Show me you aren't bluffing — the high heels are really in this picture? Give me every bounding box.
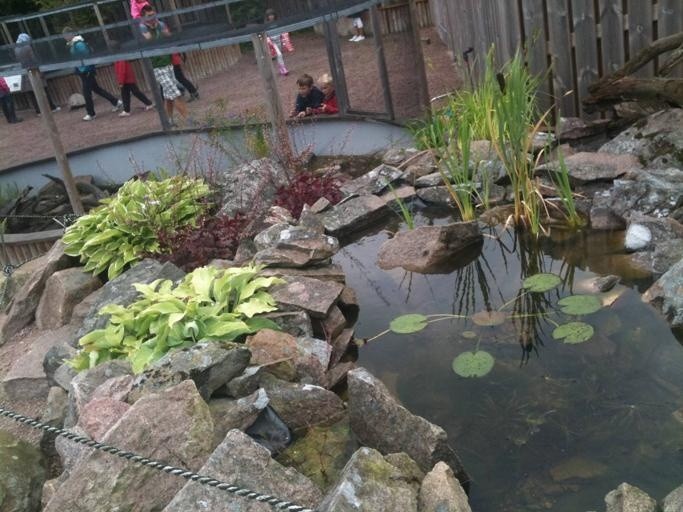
[184,91,201,103]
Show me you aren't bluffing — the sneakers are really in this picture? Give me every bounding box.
[277,63,289,75]
[347,34,365,42]
[81,113,97,121]
[110,98,124,113]
[144,101,157,111]
[118,110,131,117]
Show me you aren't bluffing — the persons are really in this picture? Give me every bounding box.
[288,73,326,118]
[140,3,191,126]
[347,12,365,41]
[130,0,168,39]
[304,73,339,114]
[62,26,122,121]
[159,53,200,101]
[0,77,23,124]
[263,8,295,74]
[14,33,62,117]
[106,40,155,117]
